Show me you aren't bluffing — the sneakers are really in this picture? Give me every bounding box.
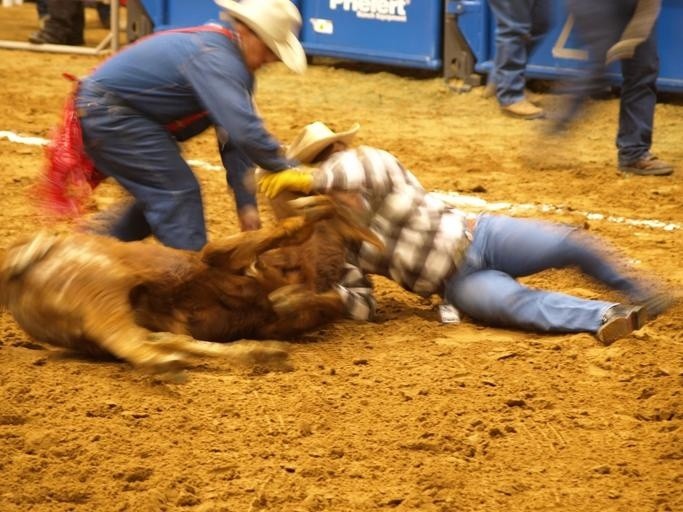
[598,302,649,344]
[27,20,84,44]
[618,149,674,176]
[501,86,546,119]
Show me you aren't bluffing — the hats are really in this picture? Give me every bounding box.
[285,121,360,167]
[214,0,308,74]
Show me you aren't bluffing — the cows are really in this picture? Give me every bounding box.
[0,168,385,384]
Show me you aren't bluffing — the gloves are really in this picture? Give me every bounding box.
[257,160,321,199]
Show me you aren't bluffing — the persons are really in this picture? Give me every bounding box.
[24,0,87,48]
[70,0,309,254]
[253,117,674,346]
[478,2,559,120]
[521,0,675,176]
